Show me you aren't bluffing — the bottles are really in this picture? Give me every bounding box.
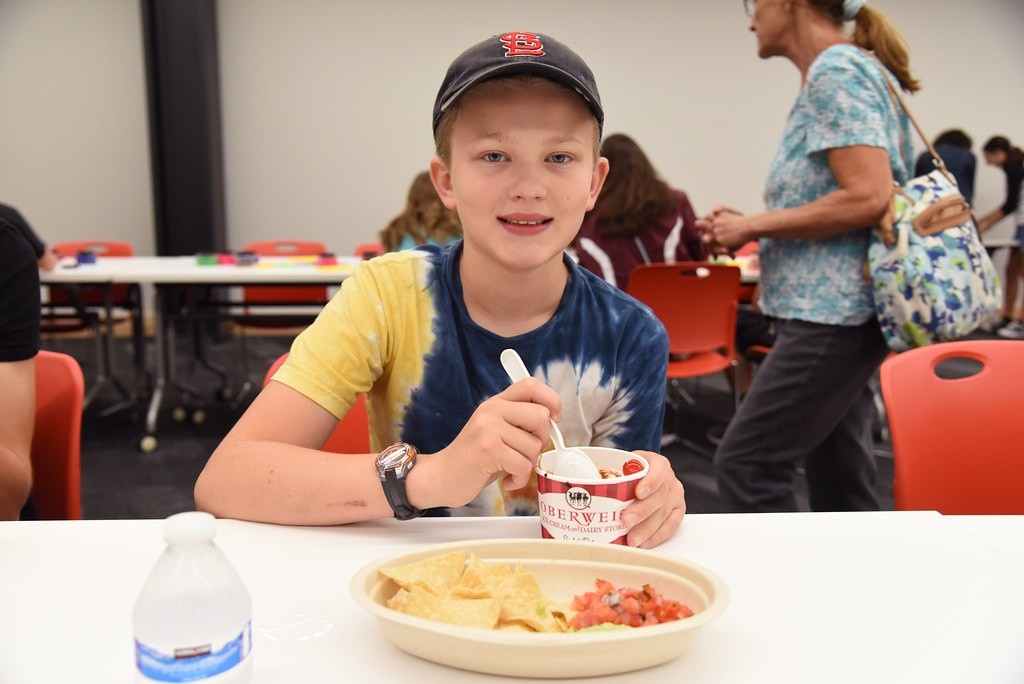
[135,512,252,684]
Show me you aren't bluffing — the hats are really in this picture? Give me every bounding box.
[432,32,604,144]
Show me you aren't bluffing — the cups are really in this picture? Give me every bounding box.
[535,442,649,546]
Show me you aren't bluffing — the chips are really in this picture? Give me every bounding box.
[380,549,632,636]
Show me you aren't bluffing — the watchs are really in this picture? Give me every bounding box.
[374,441,426,521]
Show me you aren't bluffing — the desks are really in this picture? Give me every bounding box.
[0,514,1024,684]
[709,249,762,287]
[40,256,370,451]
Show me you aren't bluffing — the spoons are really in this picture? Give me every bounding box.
[501,348,601,480]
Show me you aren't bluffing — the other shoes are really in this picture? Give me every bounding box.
[979,314,1004,332]
[998,321,1024,339]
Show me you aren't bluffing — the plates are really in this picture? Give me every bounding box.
[349,535,726,678]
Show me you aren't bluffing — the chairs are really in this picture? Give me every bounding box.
[33,240,1024,516]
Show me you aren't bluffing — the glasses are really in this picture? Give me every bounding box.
[743,0,756,18]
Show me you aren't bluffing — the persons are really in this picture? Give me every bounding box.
[0,219,42,521]
[193,30,684,548]
[694,0,923,511]
[915,128,977,207]
[577,130,708,445]
[0,203,56,271]
[974,135,1024,337]
[383,172,462,252]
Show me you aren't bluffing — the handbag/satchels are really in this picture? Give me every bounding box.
[801,43,1002,352]
